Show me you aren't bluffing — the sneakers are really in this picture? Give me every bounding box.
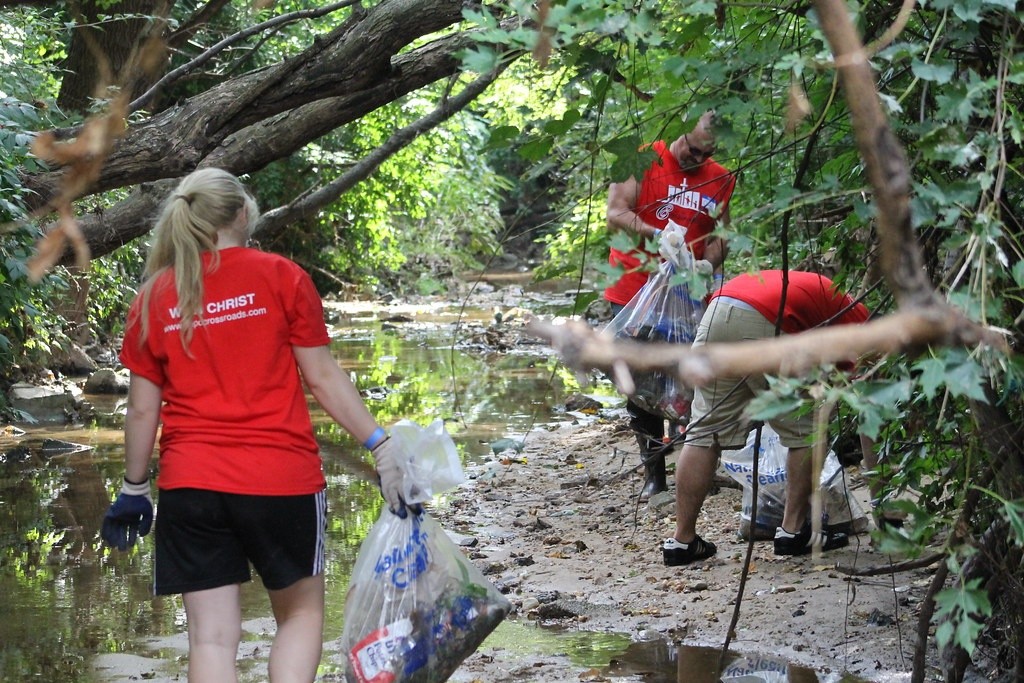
[773,521,848,555]
[663,534,716,565]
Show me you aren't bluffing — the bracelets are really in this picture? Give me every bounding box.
[651,228,662,242]
[870,499,879,505]
[364,427,385,449]
[713,275,724,279]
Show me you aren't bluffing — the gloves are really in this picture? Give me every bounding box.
[100,477,153,551]
[695,259,713,276]
[871,497,904,531]
[370,435,421,520]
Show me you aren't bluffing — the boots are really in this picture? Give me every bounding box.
[630,415,667,502]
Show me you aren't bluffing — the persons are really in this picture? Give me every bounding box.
[662,269,902,567]
[607,111,737,502]
[101,166,428,683]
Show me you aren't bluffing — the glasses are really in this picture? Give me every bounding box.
[684,133,716,158]
